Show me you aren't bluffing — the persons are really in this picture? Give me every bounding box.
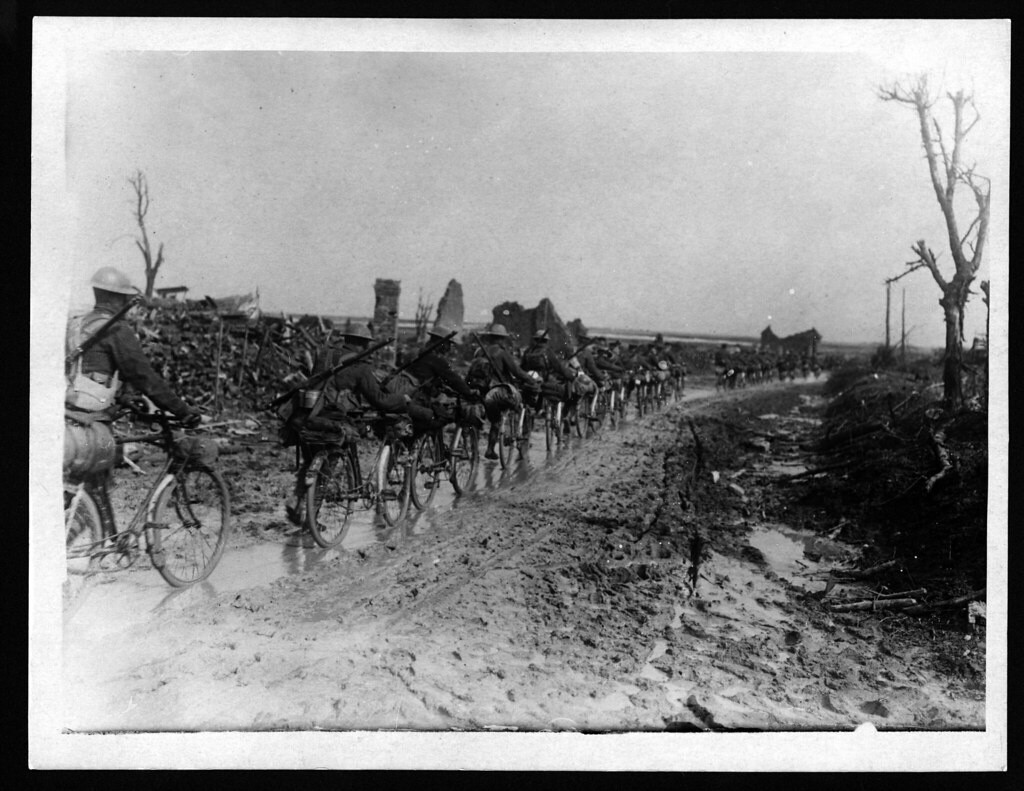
[65,265,200,588]
[285,322,411,534]
[568,327,688,418]
[516,329,584,448]
[713,342,847,390]
[464,324,544,461]
[406,324,481,469]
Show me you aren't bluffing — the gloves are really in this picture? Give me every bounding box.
[175,404,201,429]
[120,393,149,414]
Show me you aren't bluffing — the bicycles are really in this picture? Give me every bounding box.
[304,356,687,549]
[714,360,826,393]
[64,401,233,621]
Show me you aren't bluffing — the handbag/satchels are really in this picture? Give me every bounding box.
[65,373,117,414]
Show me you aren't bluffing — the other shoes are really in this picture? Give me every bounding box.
[301,518,325,532]
[484,449,497,460]
[564,417,572,433]
[286,496,305,525]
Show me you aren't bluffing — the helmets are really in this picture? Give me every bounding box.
[426,326,460,345]
[532,330,551,341]
[84,265,139,295]
[486,324,510,337]
[339,324,375,341]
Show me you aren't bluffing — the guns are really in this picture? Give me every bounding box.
[568,335,597,361]
[393,330,458,374]
[64,294,141,364]
[523,328,549,367]
[272,336,396,406]
[471,330,505,383]
